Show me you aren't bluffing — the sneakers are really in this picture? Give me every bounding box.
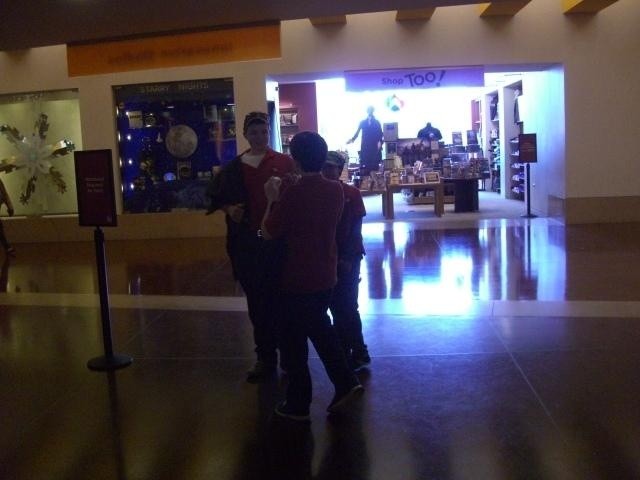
[350,350,371,371]
[275,404,311,421]
[326,384,367,413]
[246,359,278,376]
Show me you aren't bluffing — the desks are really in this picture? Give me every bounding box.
[349,171,489,220]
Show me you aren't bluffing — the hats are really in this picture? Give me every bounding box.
[243,112,271,132]
[326,150,345,169]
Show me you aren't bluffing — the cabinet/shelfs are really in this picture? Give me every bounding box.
[280,107,300,153]
[472,80,525,201]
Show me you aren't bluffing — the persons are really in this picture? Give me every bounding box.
[256,129,365,423]
[346,106,384,168]
[203,111,301,386]
[314,151,373,376]
